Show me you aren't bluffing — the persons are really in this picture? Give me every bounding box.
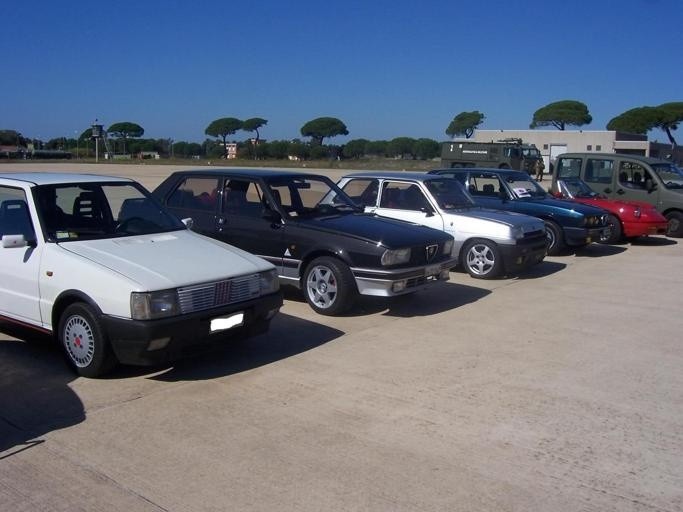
[534,159,545,183]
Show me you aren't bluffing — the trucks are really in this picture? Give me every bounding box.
[441,138,545,176]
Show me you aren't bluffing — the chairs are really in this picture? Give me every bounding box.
[0,191,107,242]
[620,172,641,185]
[168,189,281,218]
[382,187,425,210]
[483,184,494,193]
